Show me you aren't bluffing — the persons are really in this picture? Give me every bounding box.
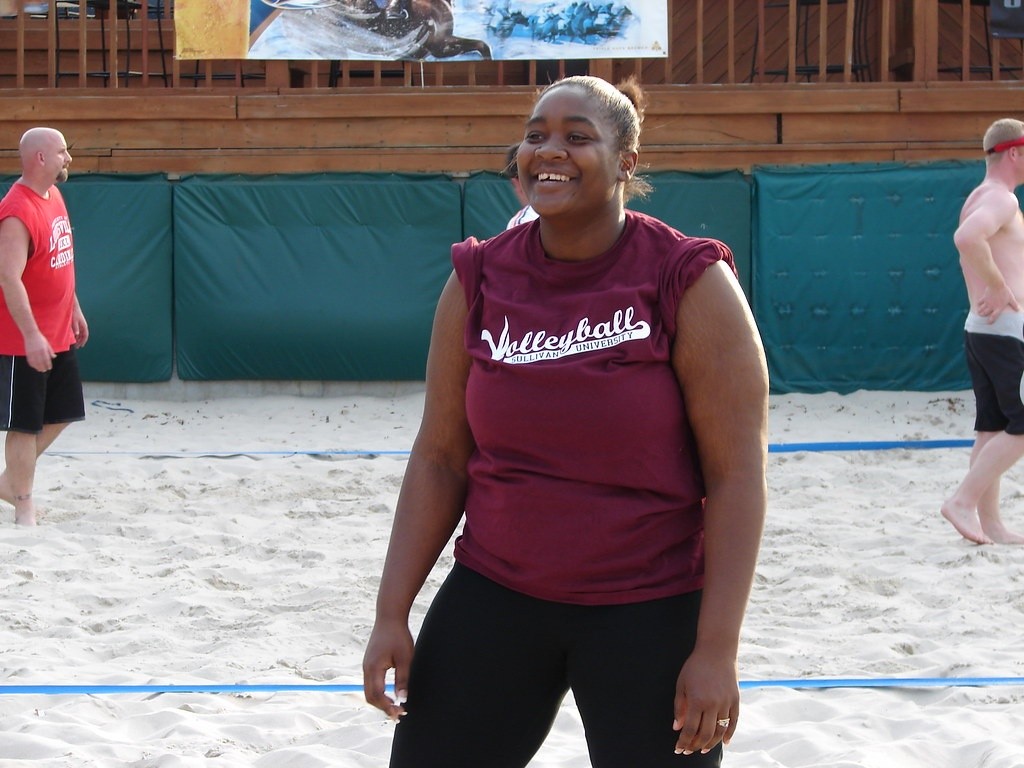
[0,127,90,528]
[940,119,1024,545]
[362,76,769,768]
[506,142,539,230]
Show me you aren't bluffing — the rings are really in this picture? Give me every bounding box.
[717,718,731,727]
